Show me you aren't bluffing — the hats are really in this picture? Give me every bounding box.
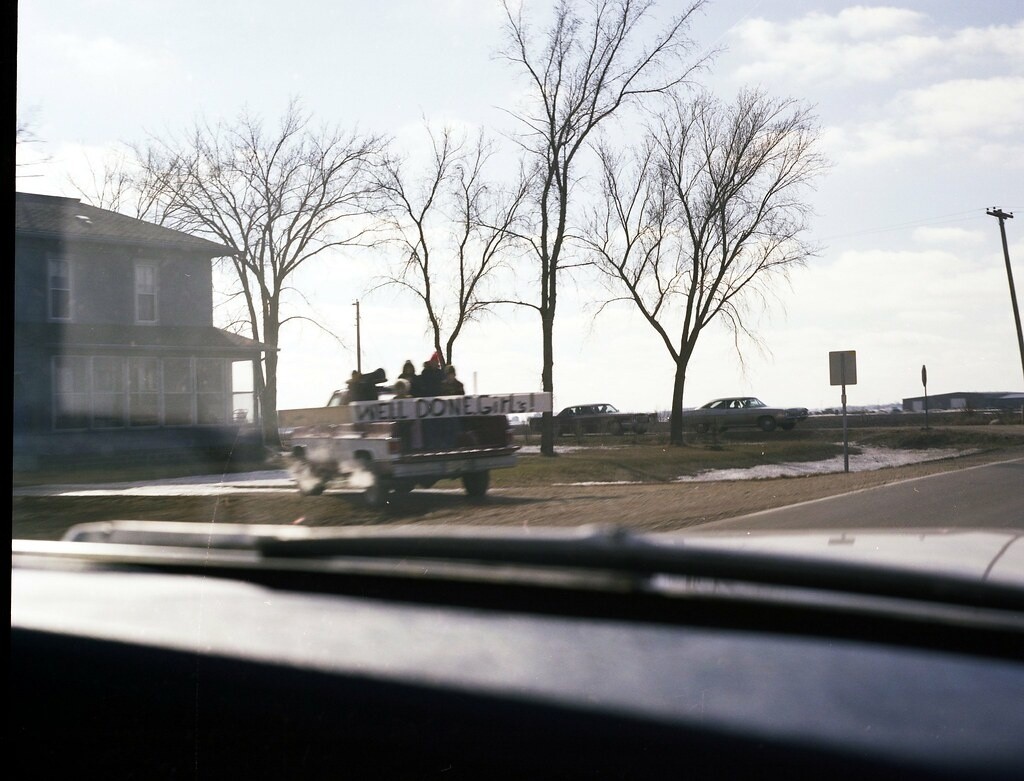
[424,360,438,370]
[443,365,456,378]
[394,379,411,395]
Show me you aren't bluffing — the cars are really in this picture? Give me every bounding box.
[667,397,810,433]
[527,404,661,437]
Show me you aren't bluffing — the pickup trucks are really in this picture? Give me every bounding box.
[290,389,522,513]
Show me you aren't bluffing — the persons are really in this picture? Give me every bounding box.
[742,401,751,408]
[734,401,740,408]
[348,369,387,405]
[391,360,466,453]
